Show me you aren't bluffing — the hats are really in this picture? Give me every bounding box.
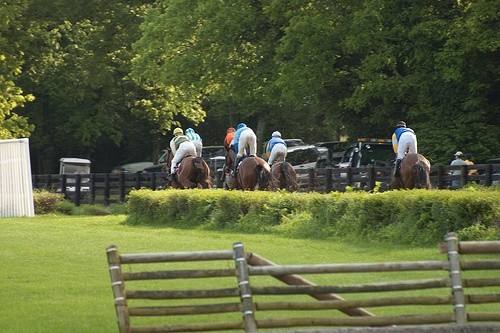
[454,152,462,156]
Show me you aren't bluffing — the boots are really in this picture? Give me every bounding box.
[231,157,241,177]
[393,159,402,177]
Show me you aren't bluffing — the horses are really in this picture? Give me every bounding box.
[225,145,280,191]
[166,147,213,189]
[391,147,431,190]
[267,158,299,193]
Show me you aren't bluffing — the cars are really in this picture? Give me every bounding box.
[207,136,399,193]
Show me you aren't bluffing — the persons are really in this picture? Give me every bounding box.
[464,154,480,184]
[168,127,197,181]
[391,121,418,177]
[231,122,258,178]
[449,150,469,188]
[224,127,236,163]
[265,130,288,167]
[185,127,203,157]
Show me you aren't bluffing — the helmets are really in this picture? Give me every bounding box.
[396,121,406,127]
[185,128,193,134]
[237,123,245,129]
[173,128,183,135]
[272,131,281,137]
[227,128,235,132]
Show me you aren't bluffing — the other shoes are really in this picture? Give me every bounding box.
[169,173,176,180]
